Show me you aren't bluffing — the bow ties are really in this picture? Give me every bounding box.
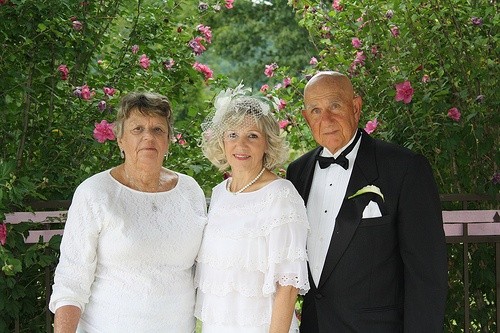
[317,128,362,171]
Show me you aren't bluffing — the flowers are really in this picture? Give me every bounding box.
[348,185,385,202]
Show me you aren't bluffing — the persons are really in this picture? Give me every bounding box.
[49,93,209,333]
[286,71,448,333]
[193,78,310,333]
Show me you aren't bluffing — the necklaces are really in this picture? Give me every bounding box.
[227,168,266,195]
[123,164,165,212]
